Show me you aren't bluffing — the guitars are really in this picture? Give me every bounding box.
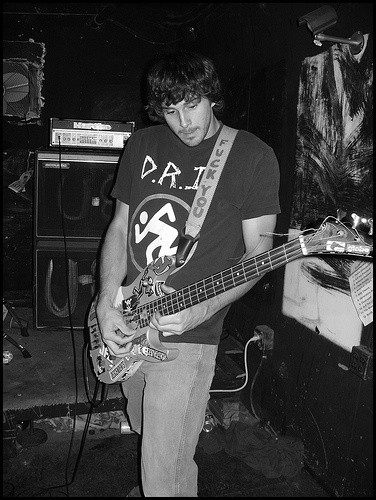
[88,209,373,384]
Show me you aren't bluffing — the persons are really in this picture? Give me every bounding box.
[96,49,281,497]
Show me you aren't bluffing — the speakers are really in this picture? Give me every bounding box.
[32,243,105,332]
[34,150,122,242]
